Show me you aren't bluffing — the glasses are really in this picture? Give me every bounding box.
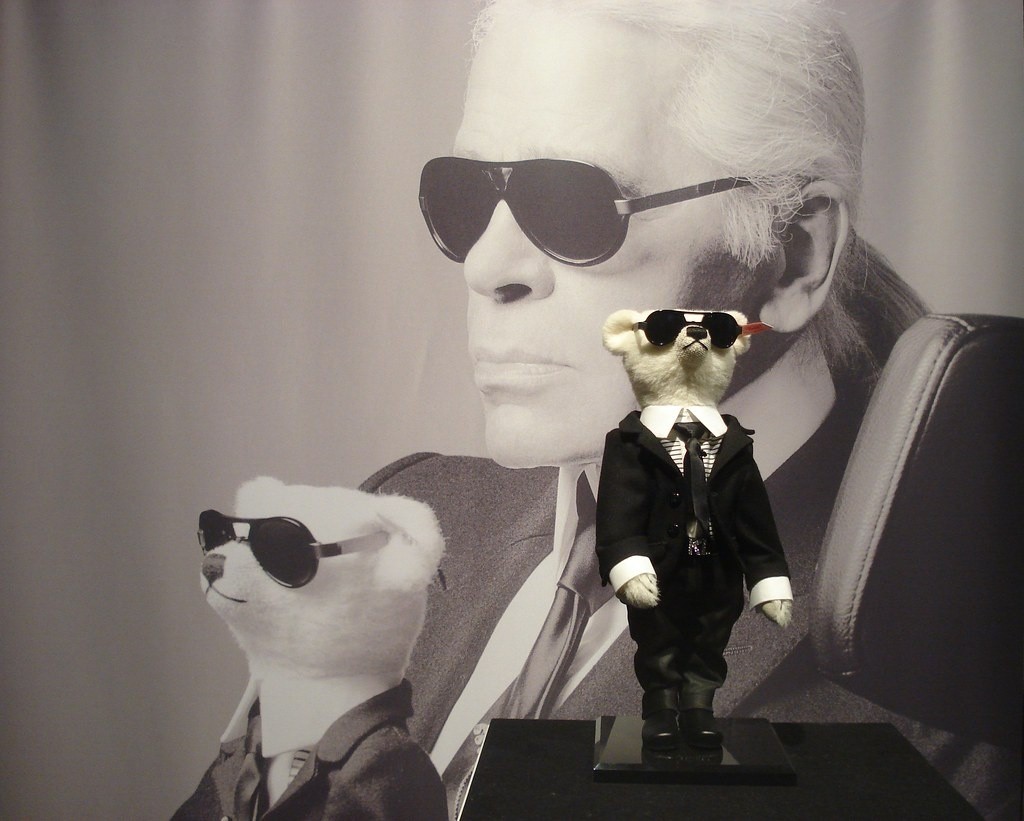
[630,308,741,348]
[419,155,758,268]
[197,510,388,589]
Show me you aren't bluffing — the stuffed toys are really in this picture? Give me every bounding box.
[199,475,451,820]
[594,309,795,751]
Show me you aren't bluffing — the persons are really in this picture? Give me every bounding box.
[164,0,1024,821]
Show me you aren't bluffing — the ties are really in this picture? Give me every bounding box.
[675,420,707,530]
[438,473,614,820]
